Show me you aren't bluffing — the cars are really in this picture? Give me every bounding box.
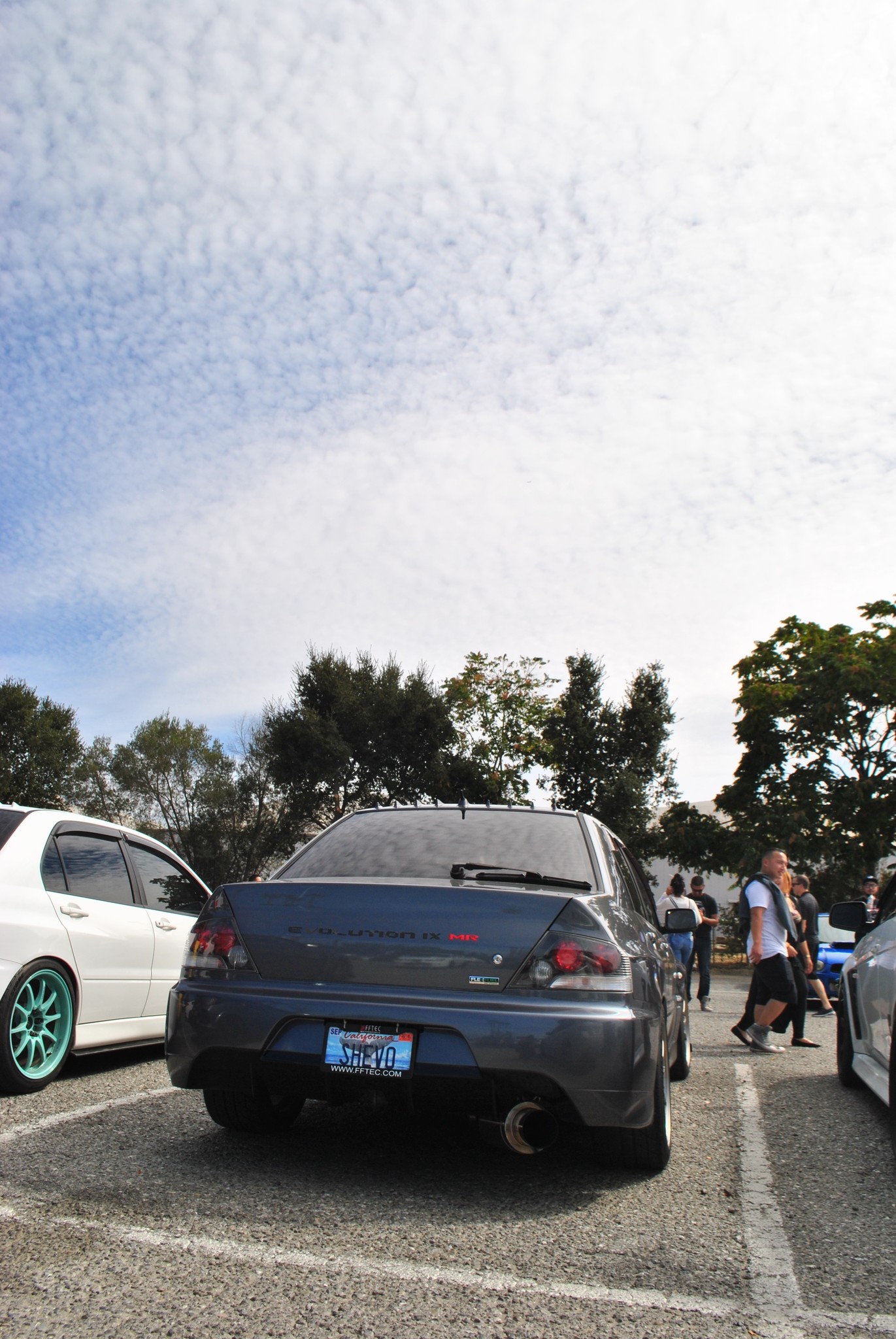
[829,869,896,1108]
[0,801,213,1091]
[807,912,856,1001]
[165,797,697,1170]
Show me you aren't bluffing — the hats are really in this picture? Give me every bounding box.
[863,875,878,885]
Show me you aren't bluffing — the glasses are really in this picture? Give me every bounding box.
[691,885,704,893]
[792,882,800,887]
[863,883,877,888]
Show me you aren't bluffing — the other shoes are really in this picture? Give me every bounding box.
[700,996,712,1011]
[731,1025,752,1046]
[791,1038,821,1047]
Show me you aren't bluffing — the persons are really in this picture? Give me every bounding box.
[684,875,719,1010]
[655,873,703,963]
[791,874,835,1017]
[854,876,885,947]
[731,848,822,1053]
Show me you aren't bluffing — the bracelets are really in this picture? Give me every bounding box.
[805,955,811,958]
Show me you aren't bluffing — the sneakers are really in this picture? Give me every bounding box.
[812,1007,836,1017]
[749,1042,786,1052]
[746,1022,782,1054]
